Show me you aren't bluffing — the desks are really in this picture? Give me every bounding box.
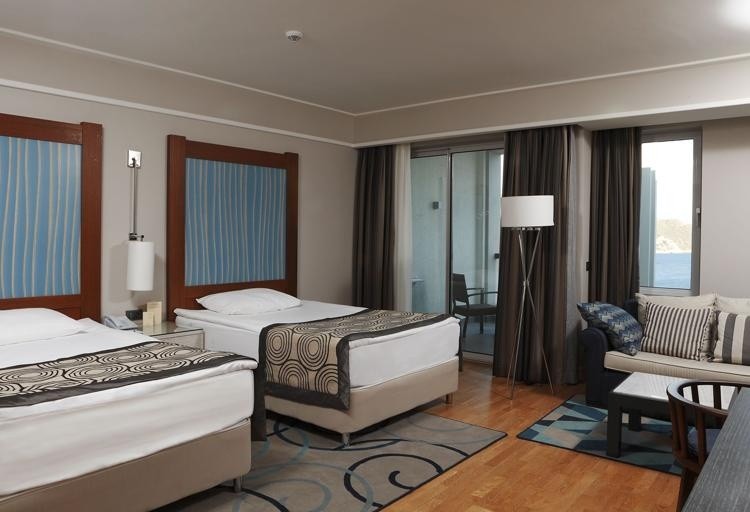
[680,386,750,512]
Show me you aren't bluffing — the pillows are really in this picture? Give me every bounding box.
[1,306,89,346]
[710,311,750,368]
[195,286,302,316]
[580,294,643,357]
[714,296,750,316]
[641,300,715,364]
[635,292,715,330]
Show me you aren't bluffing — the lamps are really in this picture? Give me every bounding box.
[498,192,558,405]
[125,148,158,293]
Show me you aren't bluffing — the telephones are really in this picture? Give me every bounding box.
[104,315,138,330]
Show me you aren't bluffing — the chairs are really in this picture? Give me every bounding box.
[665,374,750,512]
[447,270,501,337]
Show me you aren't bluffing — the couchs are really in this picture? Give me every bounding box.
[573,288,750,425]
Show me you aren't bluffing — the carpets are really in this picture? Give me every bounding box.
[516,388,693,477]
[158,394,508,512]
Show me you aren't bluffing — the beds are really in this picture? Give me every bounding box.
[0,112,261,512]
[165,132,462,446]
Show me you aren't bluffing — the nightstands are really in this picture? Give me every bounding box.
[124,318,208,350]
